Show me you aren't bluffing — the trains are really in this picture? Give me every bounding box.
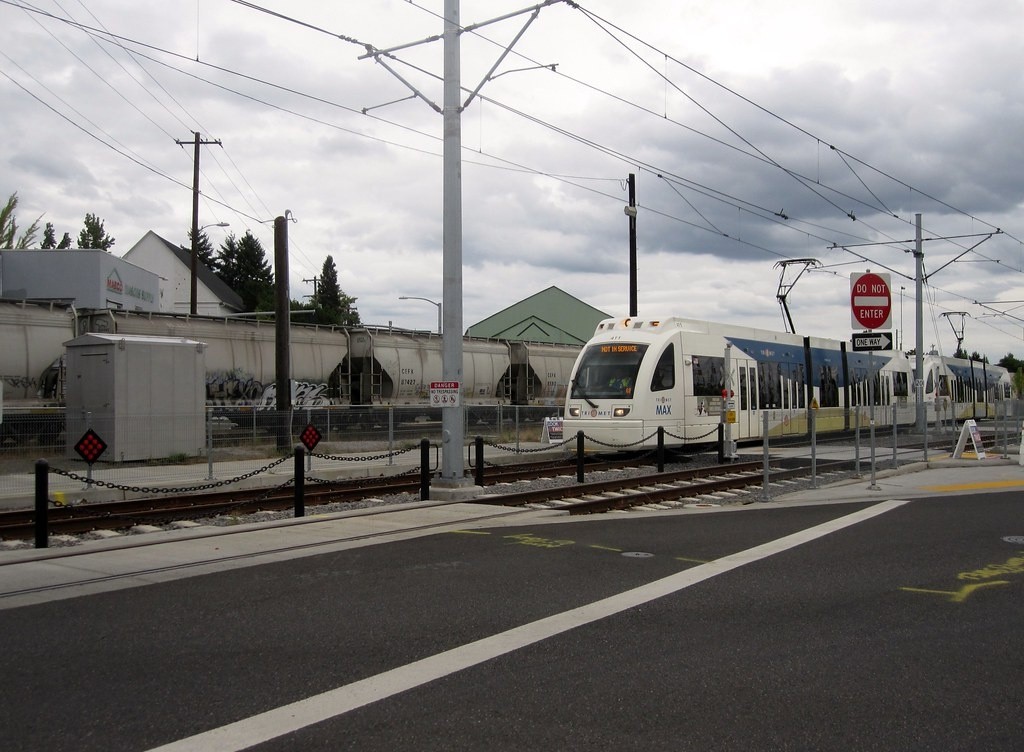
[560,315,1014,455]
[2,289,584,447]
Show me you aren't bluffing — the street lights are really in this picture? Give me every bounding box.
[397,295,443,334]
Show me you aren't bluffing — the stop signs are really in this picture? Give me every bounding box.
[848,271,895,331]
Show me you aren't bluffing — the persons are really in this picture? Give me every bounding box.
[607,366,633,391]
